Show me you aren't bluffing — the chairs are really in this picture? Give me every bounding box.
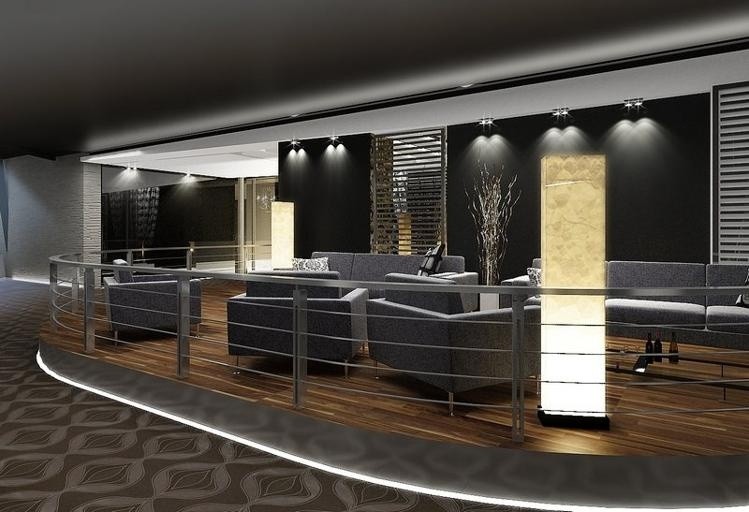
[102,256,205,346]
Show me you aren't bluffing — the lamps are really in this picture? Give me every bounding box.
[539,153,608,428]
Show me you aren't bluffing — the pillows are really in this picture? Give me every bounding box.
[290,255,329,273]
[526,265,542,298]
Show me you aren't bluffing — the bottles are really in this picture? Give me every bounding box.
[653,338,664,363]
[645,331,655,365]
[668,331,680,365]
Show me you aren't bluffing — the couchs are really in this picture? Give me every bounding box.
[499,274,538,310]
[499,256,749,351]
[273,252,479,315]
[429,272,479,312]
[366,272,539,416]
[230,269,369,378]
